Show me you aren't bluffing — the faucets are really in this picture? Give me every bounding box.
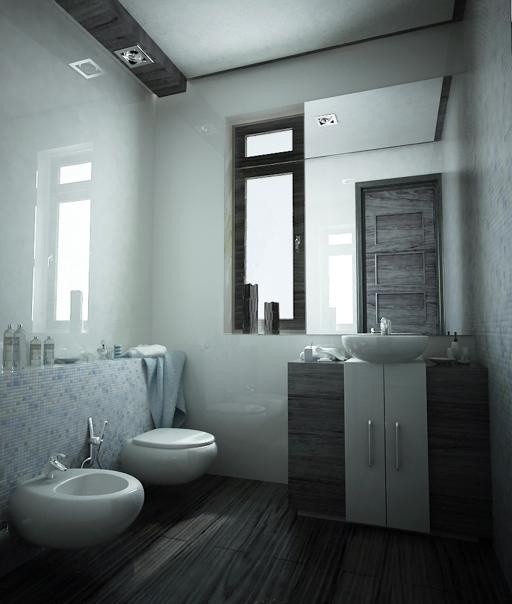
[380,317,392,335]
[44,453,67,477]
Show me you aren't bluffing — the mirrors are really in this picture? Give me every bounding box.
[303,74,467,336]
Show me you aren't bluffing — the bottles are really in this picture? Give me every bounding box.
[97,345,114,360]
[3,323,54,371]
[448,332,472,362]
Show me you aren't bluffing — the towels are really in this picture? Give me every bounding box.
[121,343,189,428]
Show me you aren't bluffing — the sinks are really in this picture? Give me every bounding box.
[342,333,429,363]
[14,469,144,548]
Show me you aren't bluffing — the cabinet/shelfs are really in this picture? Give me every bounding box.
[286,362,495,548]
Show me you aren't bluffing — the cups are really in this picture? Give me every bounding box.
[115,345,123,358]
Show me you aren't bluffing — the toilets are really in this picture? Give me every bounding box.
[120,427,217,484]
